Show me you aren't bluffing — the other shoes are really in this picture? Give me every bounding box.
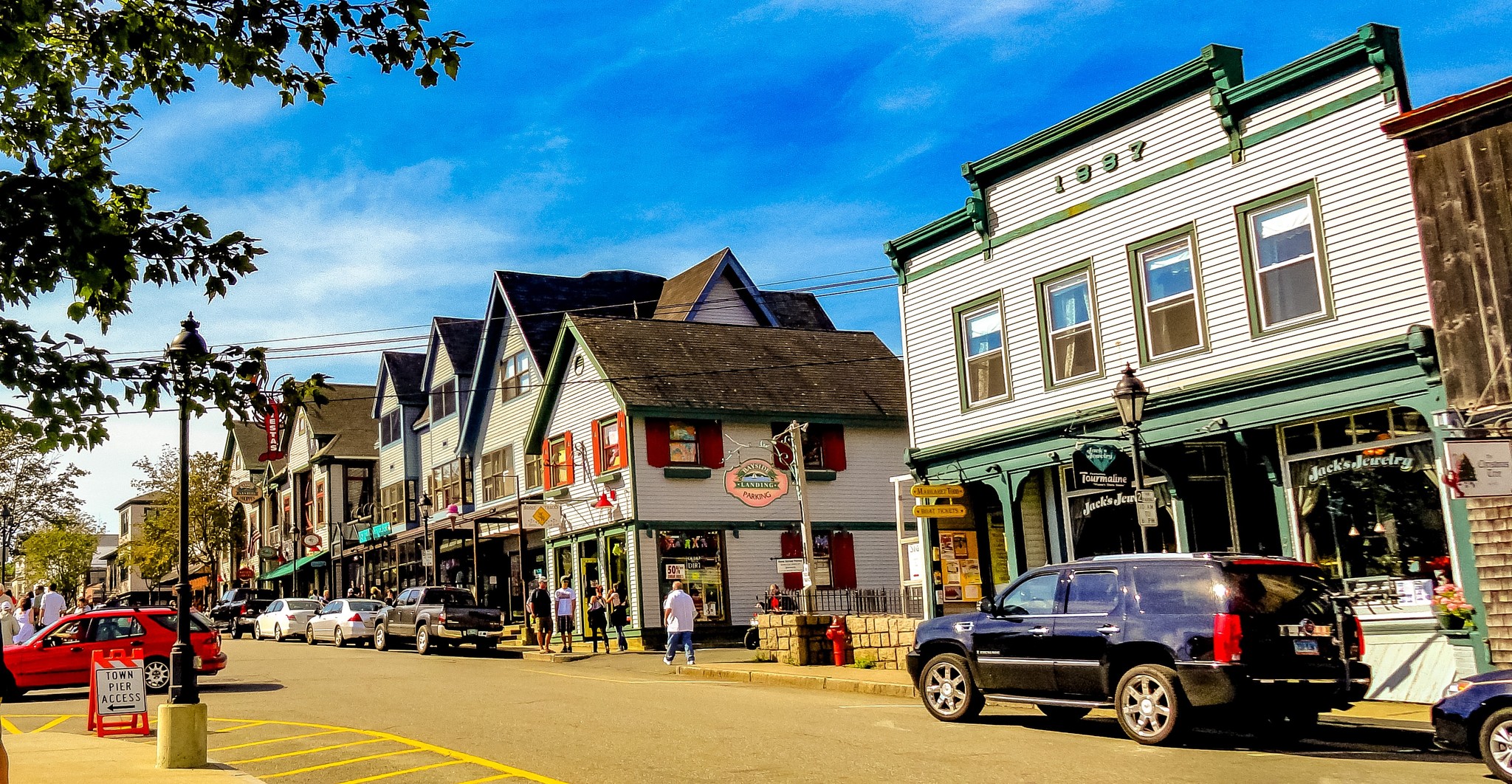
[606,649,609,654]
[618,642,627,651]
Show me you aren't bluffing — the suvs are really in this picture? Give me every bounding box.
[905,552,1373,747]
[208,586,280,639]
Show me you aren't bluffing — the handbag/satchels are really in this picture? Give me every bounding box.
[589,605,606,625]
[610,607,626,625]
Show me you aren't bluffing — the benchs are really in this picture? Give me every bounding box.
[1341,575,1393,602]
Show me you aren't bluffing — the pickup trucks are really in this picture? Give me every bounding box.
[374,584,506,656]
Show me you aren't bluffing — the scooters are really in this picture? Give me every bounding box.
[743,594,783,650]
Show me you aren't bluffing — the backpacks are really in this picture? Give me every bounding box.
[526,589,550,615]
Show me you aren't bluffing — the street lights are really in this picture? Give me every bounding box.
[416,491,434,586]
[0,506,13,586]
[164,309,208,705]
[211,544,223,610]
[288,523,300,598]
[100,582,107,602]
[1111,361,1151,554]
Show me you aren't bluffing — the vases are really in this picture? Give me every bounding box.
[1435,615,1464,630]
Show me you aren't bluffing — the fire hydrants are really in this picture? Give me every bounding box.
[826,614,849,667]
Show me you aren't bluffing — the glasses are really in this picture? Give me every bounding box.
[562,580,569,583]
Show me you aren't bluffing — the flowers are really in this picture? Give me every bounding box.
[1430,583,1476,628]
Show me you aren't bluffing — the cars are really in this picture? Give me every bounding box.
[305,597,388,648]
[254,597,328,643]
[0,606,228,704]
[1430,668,1512,784]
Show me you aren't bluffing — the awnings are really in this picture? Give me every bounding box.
[256,550,329,581]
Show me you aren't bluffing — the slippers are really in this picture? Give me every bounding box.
[540,649,556,653]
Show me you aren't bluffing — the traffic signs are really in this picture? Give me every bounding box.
[96,667,148,716]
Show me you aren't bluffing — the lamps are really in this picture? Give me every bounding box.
[1373,490,1385,533]
[1348,505,1360,538]
[595,485,617,508]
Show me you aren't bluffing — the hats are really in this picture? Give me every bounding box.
[348,588,354,592]
[538,576,549,583]
[560,574,571,584]
[33,586,46,590]
[1,601,12,610]
[77,596,85,603]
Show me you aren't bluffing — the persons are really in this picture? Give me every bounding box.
[663,581,698,665]
[529,576,556,654]
[348,579,361,596]
[370,591,379,600]
[321,589,333,607]
[39,583,67,629]
[588,585,610,654]
[384,589,394,602]
[309,589,319,600]
[756,584,796,614]
[0,584,45,645]
[346,588,357,598]
[605,583,628,652]
[554,575,576,653]
[357,584,364,598]
[74,597,91,614]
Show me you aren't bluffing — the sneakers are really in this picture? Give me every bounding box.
[687,660,694,665]
[663,656,672,665]
[560,645,567,653]
[568,646,572,652]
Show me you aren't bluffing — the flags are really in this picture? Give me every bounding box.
[248,522,261,557]
